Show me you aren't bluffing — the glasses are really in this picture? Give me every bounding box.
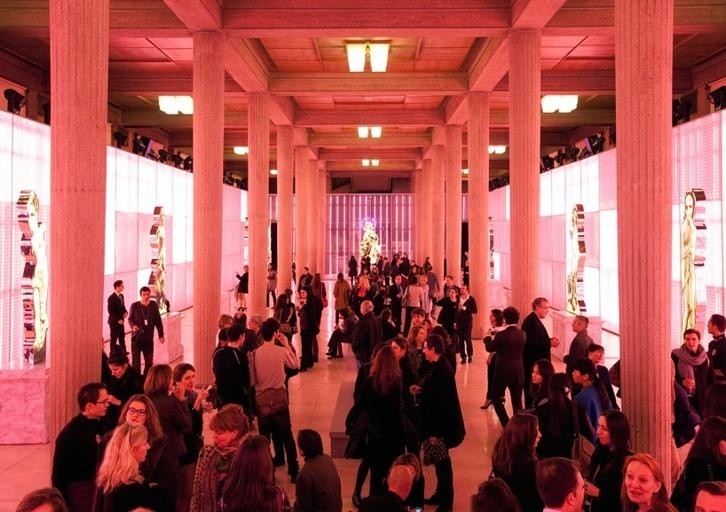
[129,408,146,416]
[96,399,109,404]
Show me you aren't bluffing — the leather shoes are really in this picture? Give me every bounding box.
[424,495,453,512]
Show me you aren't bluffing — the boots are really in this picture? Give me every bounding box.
[326,347,336,357]
[352,464,368,508]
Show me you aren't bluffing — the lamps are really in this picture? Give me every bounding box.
[342,40,393,73]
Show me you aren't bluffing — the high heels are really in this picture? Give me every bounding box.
[501,398,505,405]
[237,307,247,312]
[481,399,495,409]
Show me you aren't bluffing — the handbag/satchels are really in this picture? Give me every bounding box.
[572,433,596,479]
[279,322,292,333]
[400,400,416,433]
[422,437,448,466]
[437,308,447,324]
[323,299,328,307]
[401,294,410,308]
[255,387,287,416]
[344,428,367,459]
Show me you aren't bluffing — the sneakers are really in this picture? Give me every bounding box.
[461,355,466,364]
[468,355,473,363]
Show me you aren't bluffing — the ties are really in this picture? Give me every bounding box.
[119,294,122,305]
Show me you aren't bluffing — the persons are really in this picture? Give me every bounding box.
[101,261,343,512]
[16,487,71,512]
[93,422,159,512]
[469,297,726,512]
[97,391,179,512]
[51,380,110,512]
[324,254,478,512]
